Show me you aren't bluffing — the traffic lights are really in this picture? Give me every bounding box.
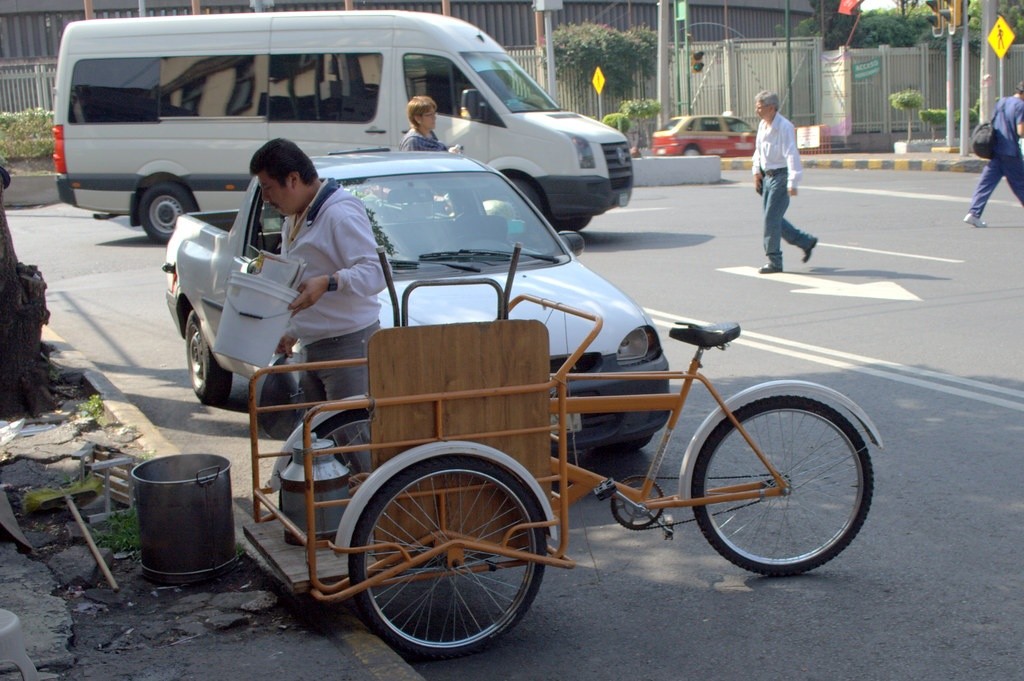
[690,48,705,73]
[925,0,944,37]
[937,0,961,35]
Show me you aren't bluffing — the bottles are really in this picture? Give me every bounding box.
[278,433,350,546]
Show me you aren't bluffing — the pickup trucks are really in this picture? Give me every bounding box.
[162,145,672,457]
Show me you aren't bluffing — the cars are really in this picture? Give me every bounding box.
[651,115,757,158]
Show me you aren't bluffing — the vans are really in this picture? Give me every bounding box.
[51,9,635,245]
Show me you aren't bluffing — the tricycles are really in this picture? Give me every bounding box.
[244,242,885,660]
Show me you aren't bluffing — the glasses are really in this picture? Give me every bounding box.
[420,112,436,117]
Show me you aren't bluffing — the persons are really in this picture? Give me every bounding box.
[963,82,1024,227]
[398,96,460,154]
[250,139,387,412]
[752,91,817,273]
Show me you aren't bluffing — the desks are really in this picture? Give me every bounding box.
[368,240,551,561]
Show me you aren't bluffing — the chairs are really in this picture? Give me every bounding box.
[471,215,507,242]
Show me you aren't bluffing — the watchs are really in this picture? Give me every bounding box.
[328,272,337,292]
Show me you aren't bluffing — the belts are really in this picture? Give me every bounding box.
[764,167,788,176]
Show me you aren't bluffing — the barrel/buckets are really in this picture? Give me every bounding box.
[256,250,301,290]
[211,270,302,368]
[132,454,235,583]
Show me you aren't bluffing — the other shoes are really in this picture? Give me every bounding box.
[963,213,987,228]
[758,263,783,274]
[801,237,817,263]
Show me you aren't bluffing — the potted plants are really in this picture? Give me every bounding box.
[889,89,1000,152]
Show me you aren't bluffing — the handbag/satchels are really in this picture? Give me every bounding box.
[972,110,999,159]
